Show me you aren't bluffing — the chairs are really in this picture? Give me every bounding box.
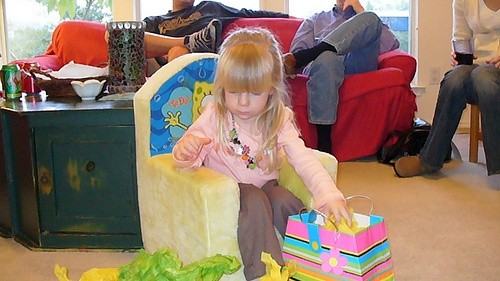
[132,52,340,281]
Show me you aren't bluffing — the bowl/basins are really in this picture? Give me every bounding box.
[71,78,107,101]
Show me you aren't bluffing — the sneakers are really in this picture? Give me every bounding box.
[183,19,222,53]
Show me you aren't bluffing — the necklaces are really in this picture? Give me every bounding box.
[229,113,282,169]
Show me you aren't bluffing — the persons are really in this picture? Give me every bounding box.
[394,0,500,177]
[106,0,297,67]
[172,27,352,281]
[282,0,400,155]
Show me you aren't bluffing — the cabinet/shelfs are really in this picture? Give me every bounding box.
[0,91,143,252]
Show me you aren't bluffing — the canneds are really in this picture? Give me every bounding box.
[1,64,22,99]
[22,62,41,94]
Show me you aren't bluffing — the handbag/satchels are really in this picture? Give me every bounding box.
[282,195,396,281]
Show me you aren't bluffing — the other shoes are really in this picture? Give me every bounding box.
[282,53,303,75]
[393,155,426,179]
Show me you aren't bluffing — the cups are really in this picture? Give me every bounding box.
[453,39,474,65]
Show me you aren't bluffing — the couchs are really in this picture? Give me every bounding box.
[7,17,416,161]
